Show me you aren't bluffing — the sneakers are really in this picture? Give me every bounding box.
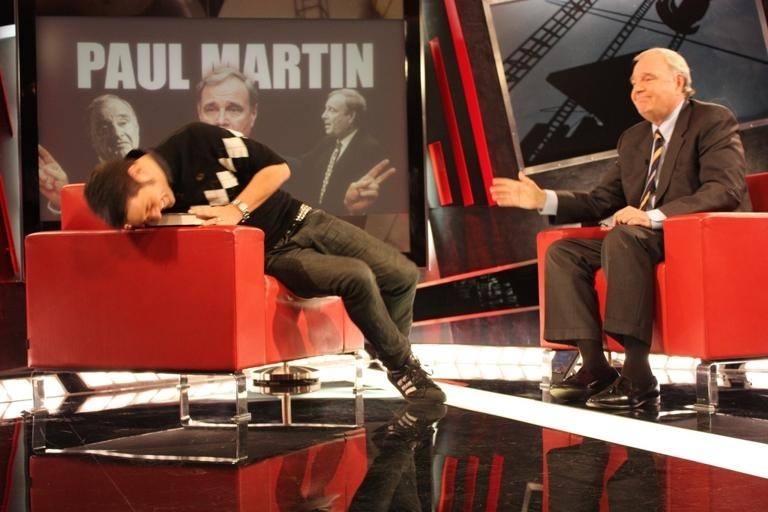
[387,354,447,405]
[373,406,448,447]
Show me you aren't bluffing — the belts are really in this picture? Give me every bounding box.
[274,202,314,252]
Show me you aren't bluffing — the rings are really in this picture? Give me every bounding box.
[216,216,225,222]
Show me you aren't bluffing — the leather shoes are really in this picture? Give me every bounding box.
[550,367,620,399]
[585,375,663,410]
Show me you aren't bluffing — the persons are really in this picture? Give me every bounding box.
[300,88,397,230]
[346,403,448,511]
[83,120,445,401]
[194,65,259,137]
[489,46,754,409]
[38,93,141,215]
[546,435,665,512]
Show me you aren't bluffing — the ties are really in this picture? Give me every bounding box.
[639,129,665,212]
[319,143,341,203]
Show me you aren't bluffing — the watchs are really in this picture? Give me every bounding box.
[229,198,250,224]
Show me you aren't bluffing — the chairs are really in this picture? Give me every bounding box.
[536,171,768,416]
[540,386,767,511]
[28,422,368,511]
[25,181,366,467]
